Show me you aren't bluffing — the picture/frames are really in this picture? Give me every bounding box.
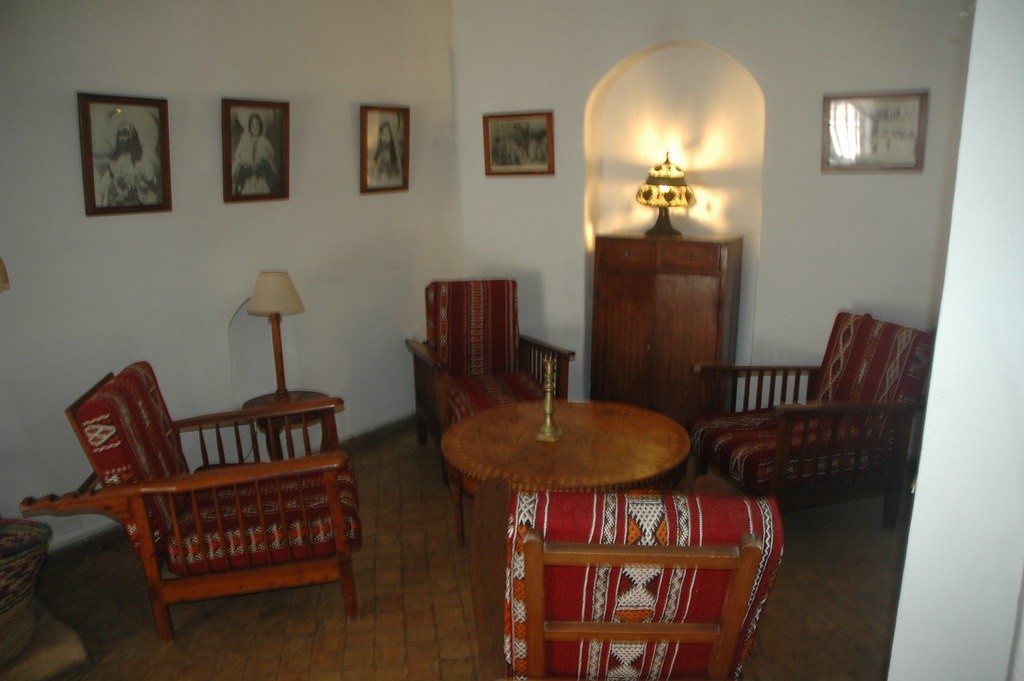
[819,87,928,175]
[358,105,411,195]
[483,115,553,175]
[79,92,172,217]
[222,98,292,202]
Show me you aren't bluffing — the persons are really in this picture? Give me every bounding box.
[235,113,275,194]
[373,124,402,184]
[102,121,158,204]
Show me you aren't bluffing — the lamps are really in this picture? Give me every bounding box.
[248,270,303,406]
[635,153,697,237]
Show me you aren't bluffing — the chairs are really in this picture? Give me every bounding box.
[498,488,783,681]
[688,311,932,530]
[19,363,365,639]
[406,282,576,489]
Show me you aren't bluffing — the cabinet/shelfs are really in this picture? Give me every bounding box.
[591,232,742,475]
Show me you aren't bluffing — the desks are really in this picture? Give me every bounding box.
[243,391,330,459]
[443,404,692,548]
